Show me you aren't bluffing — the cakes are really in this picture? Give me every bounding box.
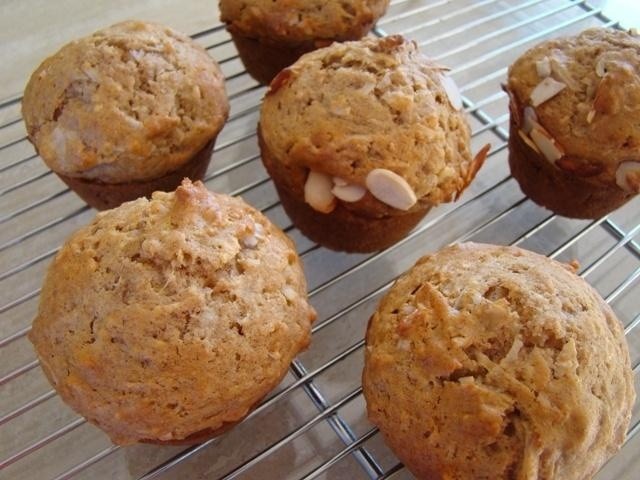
[499,29,640,219]
[219,0,389,85]
[257,36,491,251]
[28,176,317,446]
[361,241,634,479]
[22,19,230,211]
